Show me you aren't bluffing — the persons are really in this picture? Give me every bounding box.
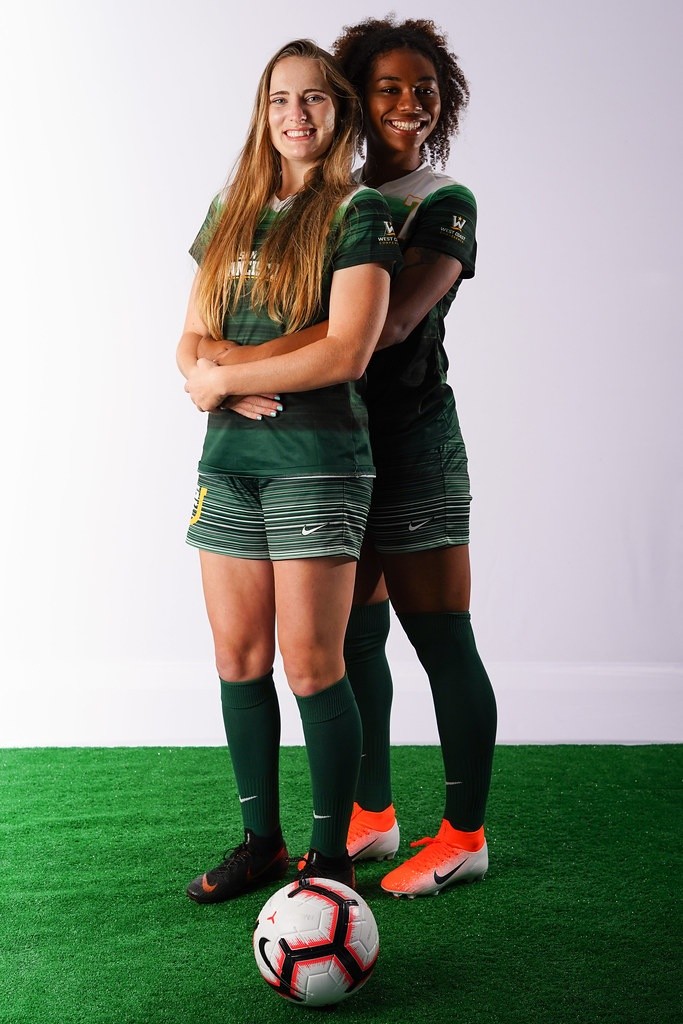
[293,15,498,895]
[175,38,399,902]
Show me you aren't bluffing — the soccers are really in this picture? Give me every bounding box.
[252,877,380,1008]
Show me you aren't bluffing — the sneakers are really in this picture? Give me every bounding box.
[298,803,399,872]
[380,819,489,899]
[294,850,355,888]
[187,841,290,903]
